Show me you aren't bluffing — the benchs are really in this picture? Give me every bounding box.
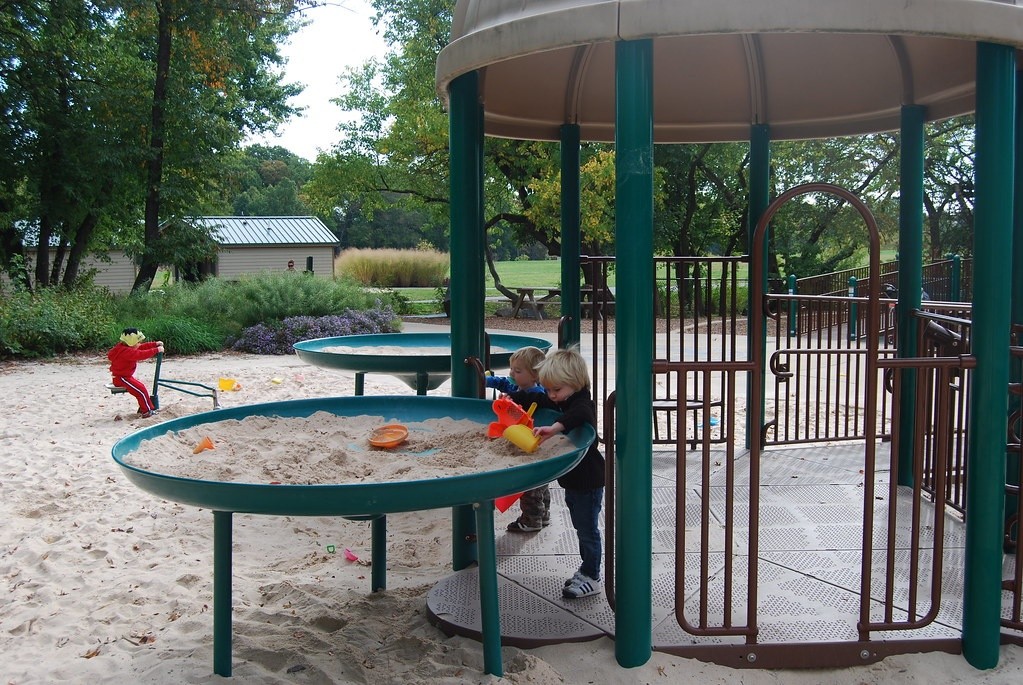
[497,299,617,320]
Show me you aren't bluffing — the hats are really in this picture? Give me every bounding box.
[120,329,145,347]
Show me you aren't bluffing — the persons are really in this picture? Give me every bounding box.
[498,348,605,598]
[108,328,165,418]
[486,345,551,532]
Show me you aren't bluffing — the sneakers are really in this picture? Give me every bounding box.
[542,516,550,526]
[562,570,601,599]
[506,515,542,531]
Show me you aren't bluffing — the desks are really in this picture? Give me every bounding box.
[505,285,611,322]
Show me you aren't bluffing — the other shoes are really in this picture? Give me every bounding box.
[142,410,158,418]
